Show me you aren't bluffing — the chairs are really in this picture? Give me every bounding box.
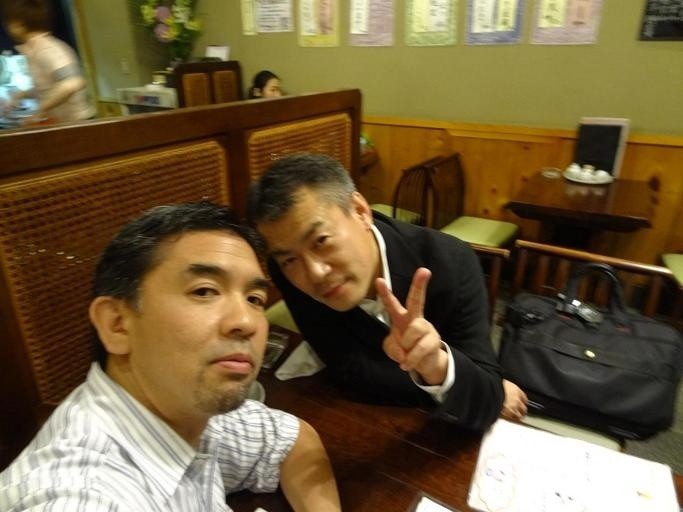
[497,238,674,453]
[661,253,683,292]
[470,243,510,324]
[421,152,521,249]
[369,155,444,225]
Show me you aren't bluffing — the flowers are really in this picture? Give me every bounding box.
[133,0,200,67]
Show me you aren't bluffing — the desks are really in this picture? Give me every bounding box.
[226,324,683,512]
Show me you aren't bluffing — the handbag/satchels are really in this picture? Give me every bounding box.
[497,255,683,442]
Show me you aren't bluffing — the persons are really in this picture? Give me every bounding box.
[0,200,342,512]
[0,0,97,135]
[247,73,280,99]
[246,151,529,438]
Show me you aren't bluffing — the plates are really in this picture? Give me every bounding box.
[562,171,612,184]
[248,381,265,403]
